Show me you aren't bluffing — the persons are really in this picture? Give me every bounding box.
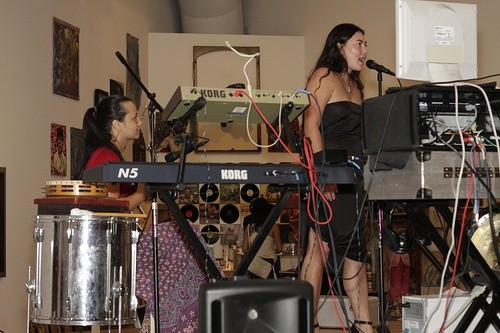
[75,95,225,333]
[243,198,281,280]
[387,224,411,303]
[301,23,375,333]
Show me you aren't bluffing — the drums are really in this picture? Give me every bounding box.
[31,195,140,327]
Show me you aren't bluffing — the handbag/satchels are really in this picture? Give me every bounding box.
[248,256,272,280]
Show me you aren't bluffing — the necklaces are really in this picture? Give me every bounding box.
[342,76,352,93]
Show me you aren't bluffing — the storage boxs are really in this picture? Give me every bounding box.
[318,297,380,329]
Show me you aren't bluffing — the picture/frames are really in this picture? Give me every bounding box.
[191,45,263,155]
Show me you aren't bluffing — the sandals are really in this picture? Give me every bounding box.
[351,320,374,333]
[313,324,320,333]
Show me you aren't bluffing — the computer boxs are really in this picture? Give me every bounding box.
[402,294,500,333]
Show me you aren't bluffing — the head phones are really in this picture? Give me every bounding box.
[383,201,417,254]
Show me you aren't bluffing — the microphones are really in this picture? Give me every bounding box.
[366,59,396,76]
[165,141,208,161]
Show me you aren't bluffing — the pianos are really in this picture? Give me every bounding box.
[80,164,356,184]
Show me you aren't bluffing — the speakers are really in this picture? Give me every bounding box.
[197,280,314,333]
